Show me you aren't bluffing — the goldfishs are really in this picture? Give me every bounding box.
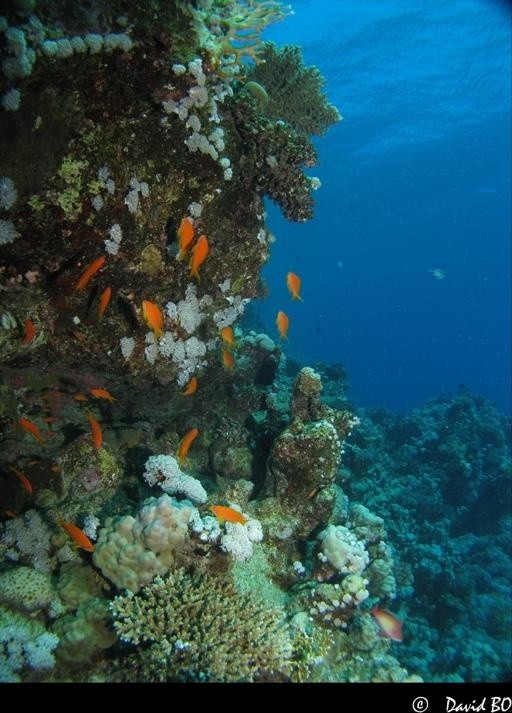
[2,210,236,499]
